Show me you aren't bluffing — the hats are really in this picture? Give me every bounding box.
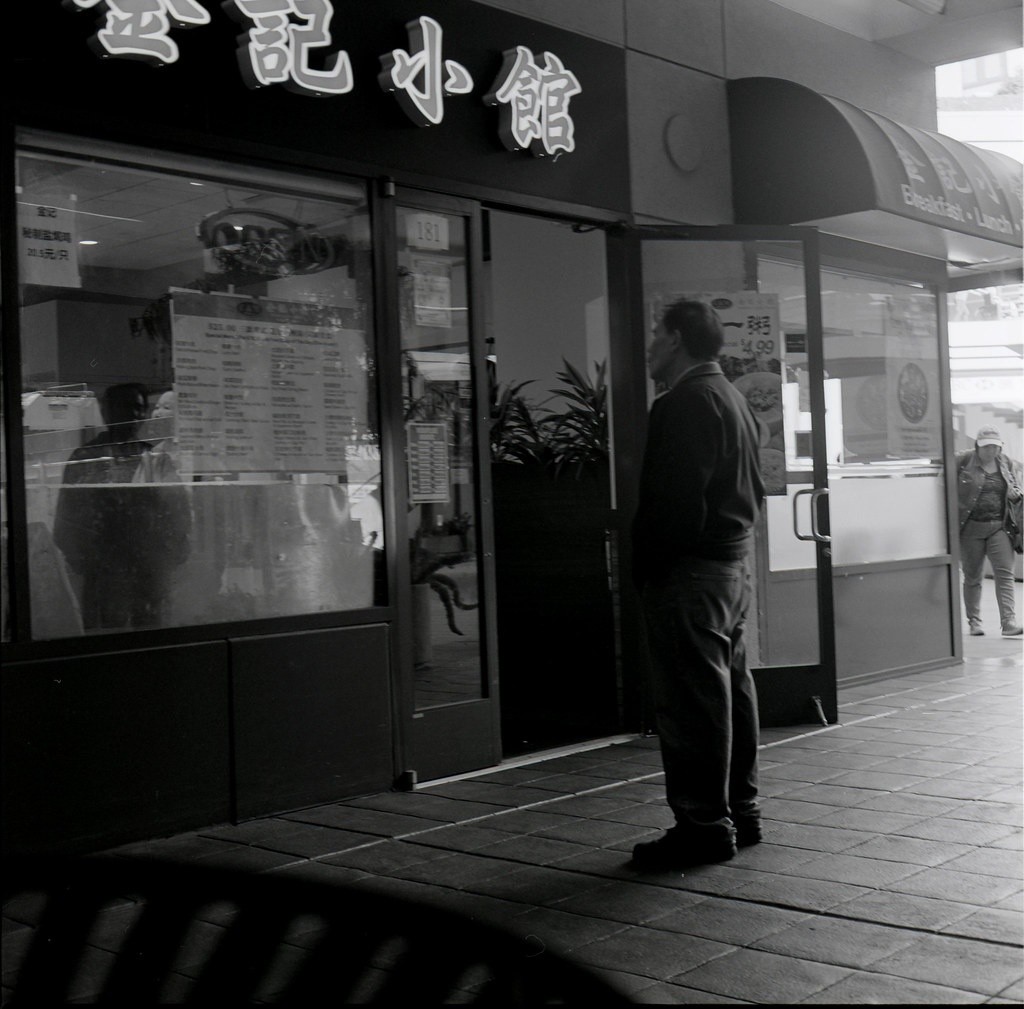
[975,424,1002,448]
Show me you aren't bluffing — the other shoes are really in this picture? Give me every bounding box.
[730,806,761,848]
[970,620,984,635]
[633,822,737,869]
[1002,625,1023,635]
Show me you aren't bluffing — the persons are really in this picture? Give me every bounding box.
[631,301,766,865]
[56,384,192,633]
[953,426,1023,635]
[130,390,193,483]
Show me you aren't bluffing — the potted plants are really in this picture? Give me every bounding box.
[433,512,474,564]
[377,527,478,672]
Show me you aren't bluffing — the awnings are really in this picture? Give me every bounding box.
[725,77,1023,284]
